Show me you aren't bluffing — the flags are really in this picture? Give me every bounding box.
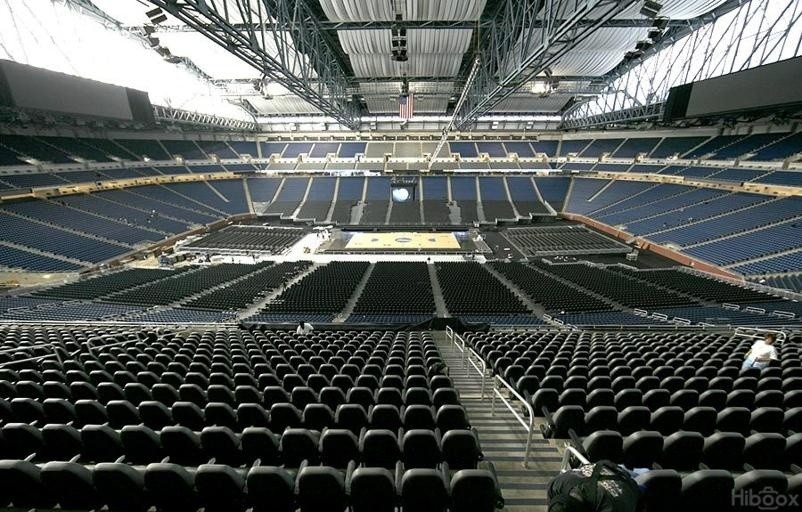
[397,92,416,119]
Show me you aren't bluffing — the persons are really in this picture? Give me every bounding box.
[181,252,257,265]
[150,213,154,218]
[314,228,331,240]
[553,255,577,264]
[147,218,151,224]
[427,257,431,263]
[740,333,780,372]
[296,320,313,335]
[547,454,650,512]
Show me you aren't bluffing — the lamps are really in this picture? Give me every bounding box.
[625,0,669,61]
[140,8,181,64]
[390,14,408,61]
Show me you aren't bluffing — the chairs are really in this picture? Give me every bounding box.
[0,129,802,512]
